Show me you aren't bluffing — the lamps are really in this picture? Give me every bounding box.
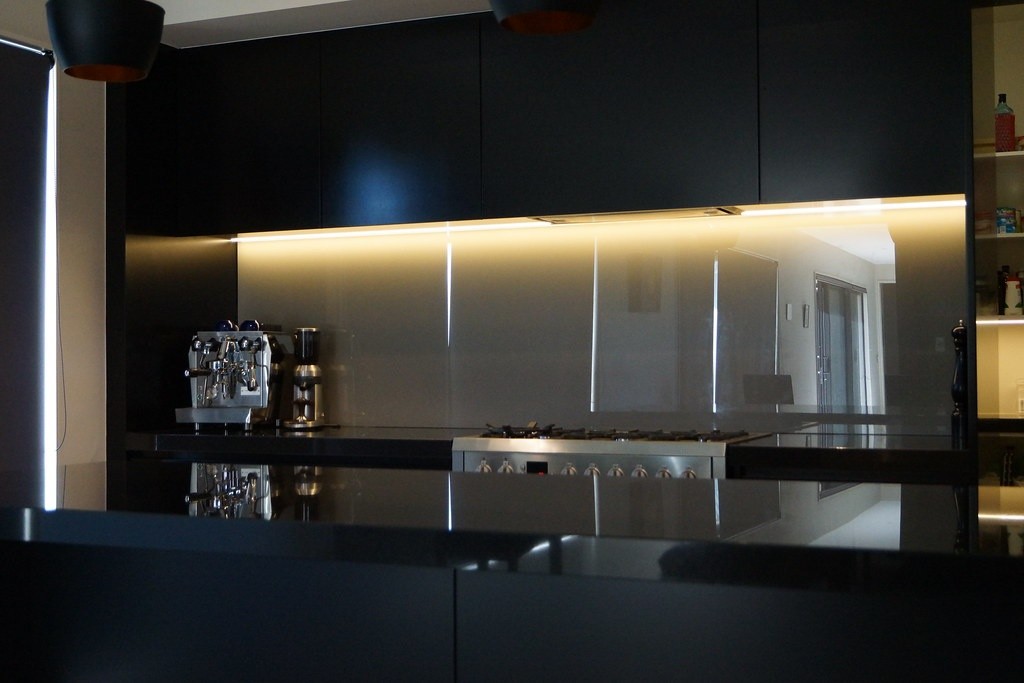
[43,0,167,86]
[487,0,604,39]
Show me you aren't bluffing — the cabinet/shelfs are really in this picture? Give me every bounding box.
[975,135,1024,559]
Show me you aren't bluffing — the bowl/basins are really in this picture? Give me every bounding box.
[216,319,233,331]
[239,320,259,331]
[233,325,239,331]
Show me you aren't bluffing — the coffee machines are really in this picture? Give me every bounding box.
[283,327,327,431]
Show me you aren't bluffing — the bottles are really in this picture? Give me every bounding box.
[995,94,1014,113]
[1000,265,1010,288]
